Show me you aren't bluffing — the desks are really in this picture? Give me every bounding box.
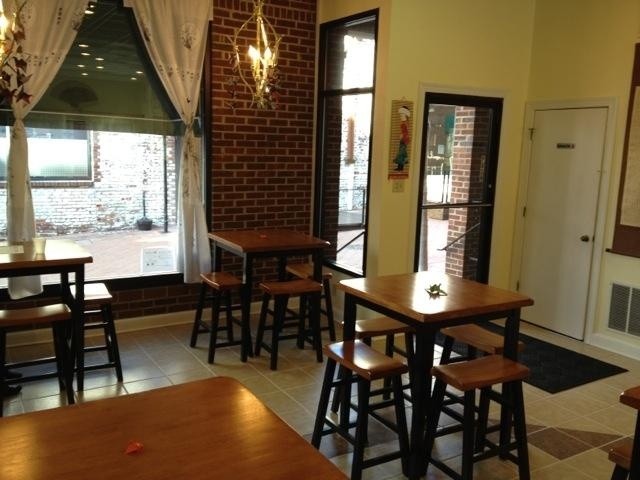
[0,238,94,393]
[329,270,536,480]
[0,376,352,480]
[207,228,330,363]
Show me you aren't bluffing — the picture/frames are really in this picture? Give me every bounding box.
[389,99,411,179]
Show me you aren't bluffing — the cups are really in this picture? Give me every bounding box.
[33,237,46,254]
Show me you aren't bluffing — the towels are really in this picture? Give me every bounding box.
[427,175,443,202]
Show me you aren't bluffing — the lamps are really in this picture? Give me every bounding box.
[225,0,286,112]
[0,0,20,93]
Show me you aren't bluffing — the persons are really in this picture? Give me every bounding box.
[391,105,410,171]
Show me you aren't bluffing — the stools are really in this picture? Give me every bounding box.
[332,316,415,422]
[255,280,324,370]
[311,339,409,480]
[607,435,638,480]
[419,354,532,480]
[189,273,248,363]
[435,323,526,452]
[68,281,124,391]
[0,298,75,404]
[285,262,336,341]
